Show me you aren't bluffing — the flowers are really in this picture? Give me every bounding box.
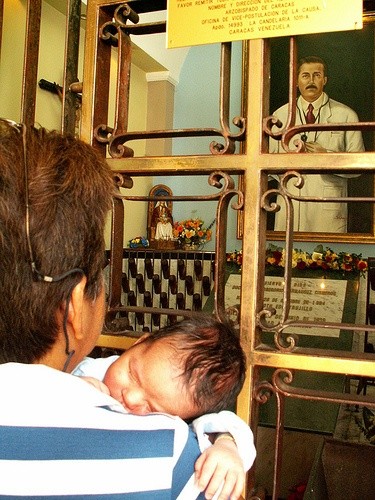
[172,218,213,251]
[127,236,150,249]
[226,243,369,279]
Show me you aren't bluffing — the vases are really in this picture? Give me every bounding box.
[184,244,200,251]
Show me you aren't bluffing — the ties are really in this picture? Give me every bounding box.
[307,104,315,124]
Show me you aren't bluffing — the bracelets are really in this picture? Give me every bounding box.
[214,436,234,444]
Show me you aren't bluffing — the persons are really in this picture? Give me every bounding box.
[0,116,257,500]
